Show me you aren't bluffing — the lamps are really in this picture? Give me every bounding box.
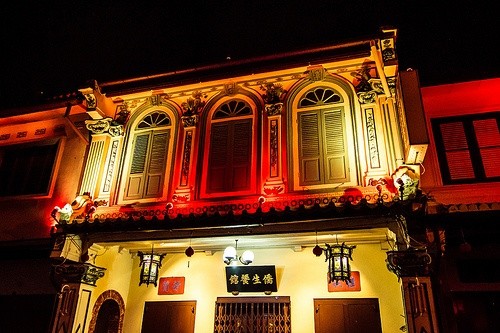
[51,206,62,225]
[164,201,173,218]
[137,243,167,287]
[375,183,384,203]
[320,232,358,286]
[223,239,255,266]
[258,195,265,211]
[395,176,405,200]
[85,206,97,223]
